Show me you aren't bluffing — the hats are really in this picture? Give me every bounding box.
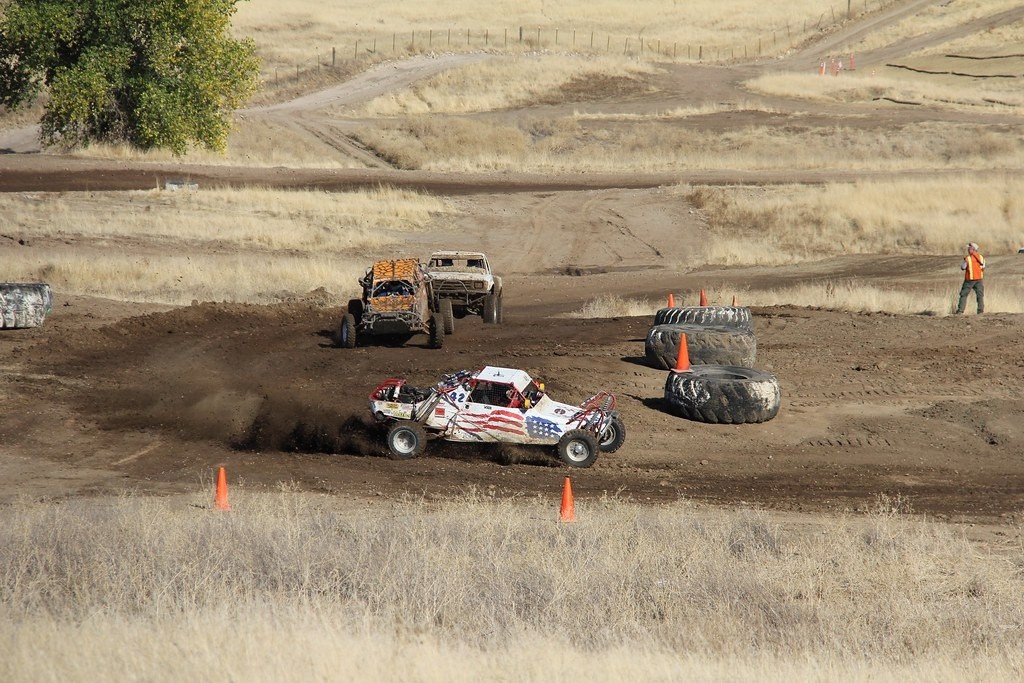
[966,243,979,251]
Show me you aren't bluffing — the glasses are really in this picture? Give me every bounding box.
[969,243,972,247]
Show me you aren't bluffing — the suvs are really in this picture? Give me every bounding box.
[368,366,627,469]
[341,258,454,348]
[423,251,504,323]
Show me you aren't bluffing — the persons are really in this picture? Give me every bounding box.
[956,243,985,314]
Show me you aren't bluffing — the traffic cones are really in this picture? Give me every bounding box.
[698,288,708,306]
[667,294,675,308]
[669,332,695,374]
[733,296,737,307]
[210,466,236,514]
[560,477,580,524]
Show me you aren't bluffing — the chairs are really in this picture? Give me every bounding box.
[479,394,503,406]
[467,260,481,267]
[442,259,453,266]
